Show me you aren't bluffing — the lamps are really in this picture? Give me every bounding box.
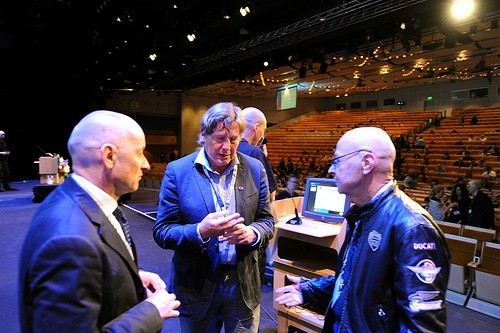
[470,24,477,36]
[402,40,411,52]
[415,36,421,46]
[300,64,307,78]
[491,20,497,31]
[319,62,328,73]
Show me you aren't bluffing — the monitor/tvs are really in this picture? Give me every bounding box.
[301,178,350,224]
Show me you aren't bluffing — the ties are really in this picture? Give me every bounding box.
[112,206,133,250]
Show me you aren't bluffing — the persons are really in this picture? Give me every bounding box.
[270,147,335,201]
[18,110,180,333]
[331,112,500,229]
[152,102,275,333]
[0,130,15,192]
[237,108,277,203]
[274,127,451,333]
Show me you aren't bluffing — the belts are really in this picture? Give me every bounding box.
[215,270,240,284]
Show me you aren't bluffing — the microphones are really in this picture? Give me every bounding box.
[281,180,302,225]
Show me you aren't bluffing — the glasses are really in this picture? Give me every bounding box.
[327,148,373,167]
